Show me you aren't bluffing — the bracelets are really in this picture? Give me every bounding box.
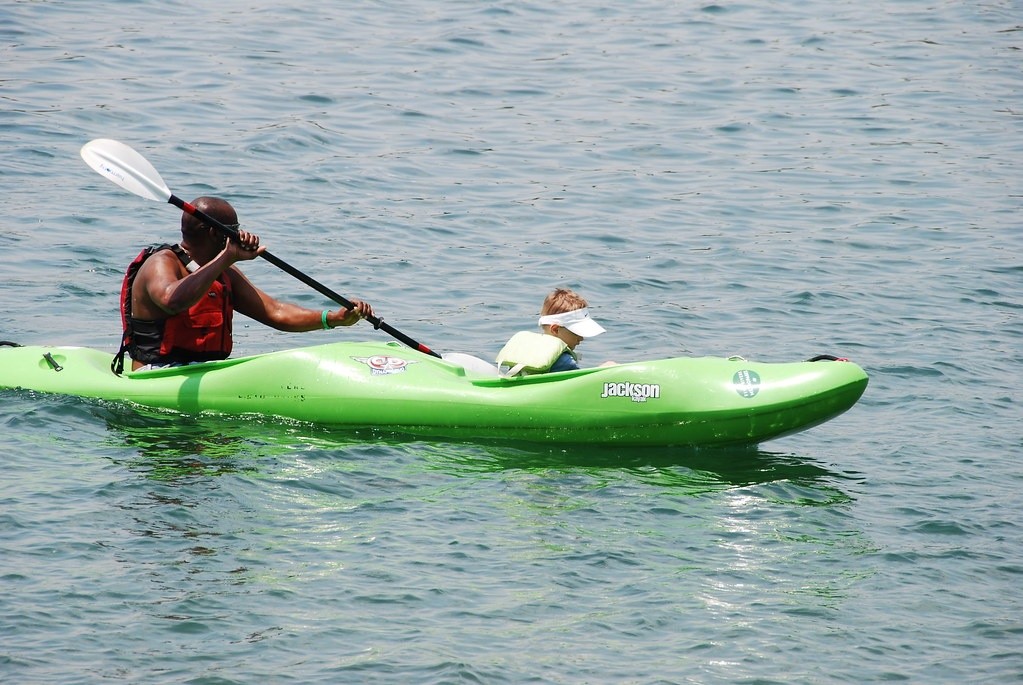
[322,309,335,330]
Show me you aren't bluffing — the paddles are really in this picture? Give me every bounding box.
[79,135,504,376]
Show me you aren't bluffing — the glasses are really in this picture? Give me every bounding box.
[200,223,240,232]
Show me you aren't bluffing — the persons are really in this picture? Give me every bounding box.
[120,196,374,372]
[509,287,620,378]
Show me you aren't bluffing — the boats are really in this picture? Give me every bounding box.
[0,334,874,453]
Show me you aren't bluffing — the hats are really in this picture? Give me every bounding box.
[538,306,607,338]
